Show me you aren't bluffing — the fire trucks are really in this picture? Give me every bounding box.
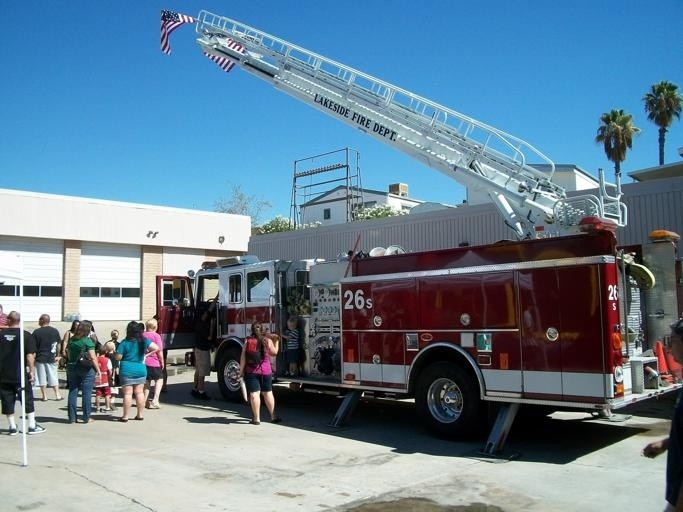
[153,10,683,464]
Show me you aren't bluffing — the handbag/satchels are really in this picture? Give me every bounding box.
[77,352,92,372]
[239,377,247,402]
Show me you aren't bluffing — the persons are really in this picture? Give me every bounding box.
[238,321,279,423]
[0,304,167,436]
[280,316,304,377]
[190,291,218,398]
[640,317,682,511]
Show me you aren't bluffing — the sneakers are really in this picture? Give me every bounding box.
[10,387,211,436]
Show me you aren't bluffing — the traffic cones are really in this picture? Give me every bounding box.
[655,340,673,386]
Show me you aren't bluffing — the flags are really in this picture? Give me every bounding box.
[159,9,197,56]
[202,31,248,73]
